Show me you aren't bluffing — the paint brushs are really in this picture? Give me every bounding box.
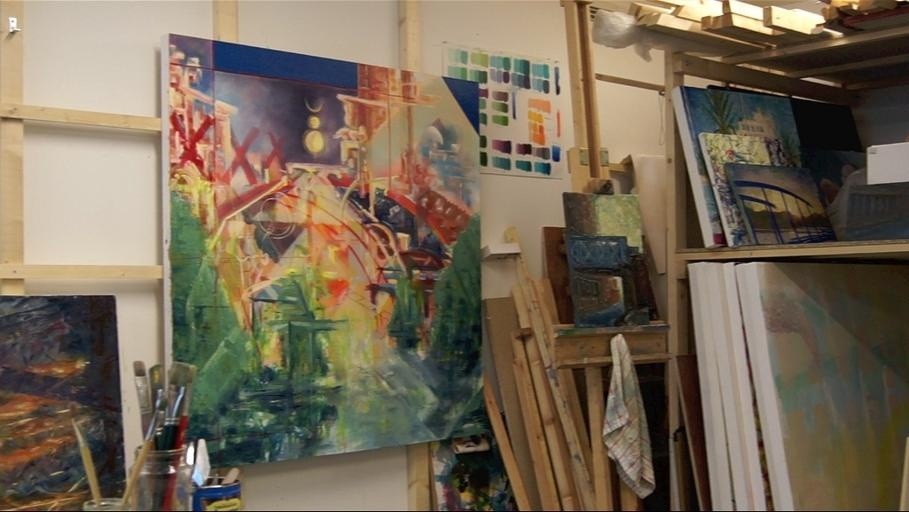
[63,357,196,512]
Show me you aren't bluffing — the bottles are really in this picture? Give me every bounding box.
[83,498,129,511]
[194,477,241,511]
[128,444,194,511]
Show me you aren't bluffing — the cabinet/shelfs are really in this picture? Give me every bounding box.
[669,33,908,512]
